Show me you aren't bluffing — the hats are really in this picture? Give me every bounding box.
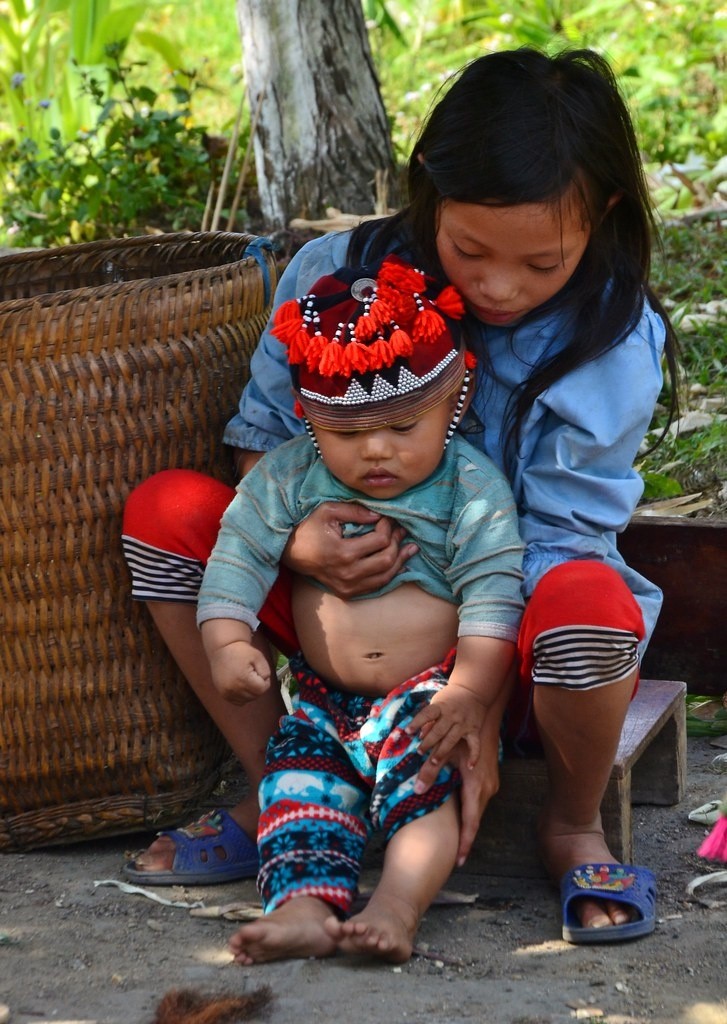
[268,252,478,432]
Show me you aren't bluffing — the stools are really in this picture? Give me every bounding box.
[502,680,688,865]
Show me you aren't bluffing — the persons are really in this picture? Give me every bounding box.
[196,252,525,965]
[122,49,678,944]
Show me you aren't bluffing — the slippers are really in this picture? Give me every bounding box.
[557,863,658,943]
[120,806,261,886]
